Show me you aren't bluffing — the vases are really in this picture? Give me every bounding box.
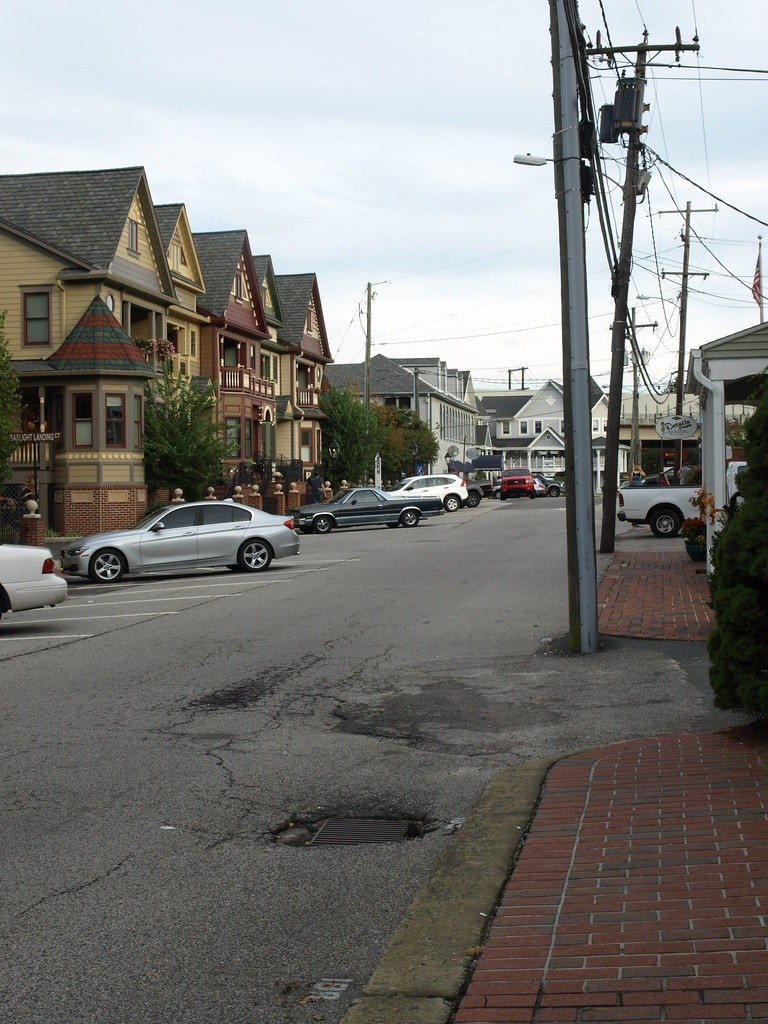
[686,543,707,561]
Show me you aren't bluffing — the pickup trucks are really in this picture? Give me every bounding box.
[617,461,749,539]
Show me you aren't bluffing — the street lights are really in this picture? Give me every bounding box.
[513,154,636,553]
[637,295,687,486]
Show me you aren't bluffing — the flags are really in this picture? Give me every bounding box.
[752,259,761,306]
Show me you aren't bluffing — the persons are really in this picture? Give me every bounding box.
[678,463,689,485]
[656,468,670,486]
[307,471,327,503]
[629,465,646,483]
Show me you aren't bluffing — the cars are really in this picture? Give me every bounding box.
[492,478,546,498]
[286,487,444,534]
[531,474,566,497]
[60,501,300,583]
[0,544,68,620]
[384,474,469,512]
[639,467,674,487]
[464,479,493,508]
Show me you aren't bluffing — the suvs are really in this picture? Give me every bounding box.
[497,468,537,500]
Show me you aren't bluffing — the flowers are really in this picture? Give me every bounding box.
[679,517,707,545]
